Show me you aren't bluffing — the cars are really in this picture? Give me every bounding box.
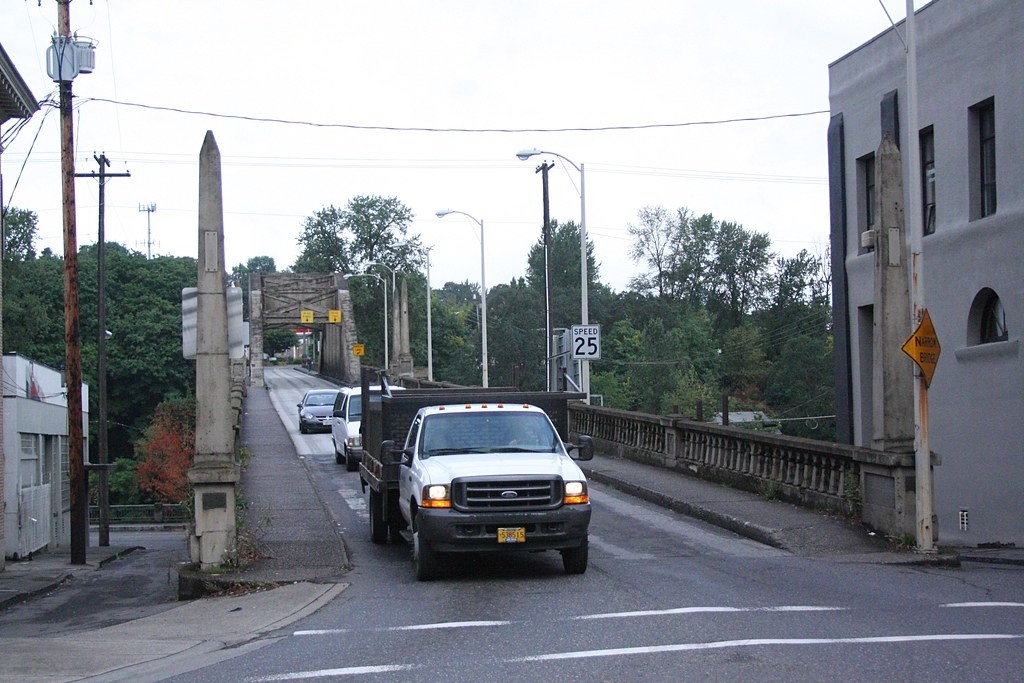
[297,390,341,435]
[713,411,782,435]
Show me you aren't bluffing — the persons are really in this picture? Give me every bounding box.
[306,356,314,372]
[509,421,531,445]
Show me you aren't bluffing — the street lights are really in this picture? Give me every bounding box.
[343,271,389,372]
[436,206,496,396]
[387,239,435,384]
[516,147,593,405]
[360,260,395,299]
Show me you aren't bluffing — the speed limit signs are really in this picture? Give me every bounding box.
[571,325,602,361]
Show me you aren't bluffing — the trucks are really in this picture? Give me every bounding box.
[354,358,597,578]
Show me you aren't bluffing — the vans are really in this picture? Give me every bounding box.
[331,384,406,473]
